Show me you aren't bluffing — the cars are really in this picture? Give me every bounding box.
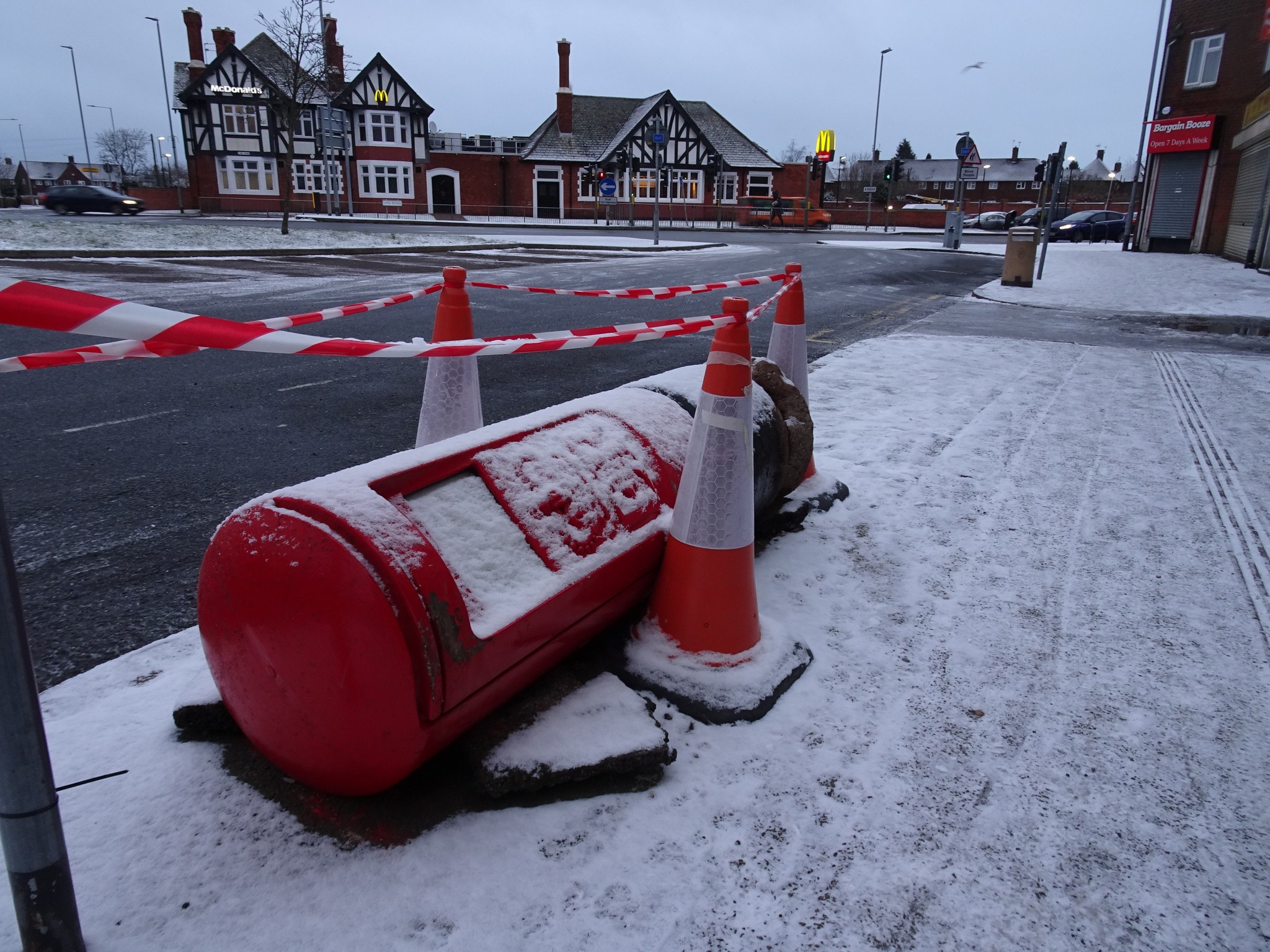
[963,214,980,221]
[977,211,1008,218]
[1123,212,1140,235]
[17,193,48,205]
[963,214,1006,230]
[1044,210,1129,243]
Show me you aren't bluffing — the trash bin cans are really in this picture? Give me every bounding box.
[1000,226,1039,289]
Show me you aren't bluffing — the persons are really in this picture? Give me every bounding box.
[763,189,784,228]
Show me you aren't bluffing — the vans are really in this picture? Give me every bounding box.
[43,185,146,216]
[738,196,832,230]
[1014,206,1072,229]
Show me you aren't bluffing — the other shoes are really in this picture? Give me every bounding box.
[763,224,769,228]
[779,226,785,230]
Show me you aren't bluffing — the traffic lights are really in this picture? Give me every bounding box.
[598,172,605,184]
[812,158,822,181]
[706,152,719,176]
[884,166,891,181]
[615,149,627,172]
[1035,163,1045,182]
[894,158,905,181]
[582,165,592,184]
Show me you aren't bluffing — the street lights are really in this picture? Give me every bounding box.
[500,155,508,216]
[62,44,98,185]
[866,47,892,228]
[145,15,186,214]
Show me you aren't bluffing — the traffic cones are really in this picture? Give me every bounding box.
[613,297,813,723]
[414,265,484,449]
[766,261,849,512]
